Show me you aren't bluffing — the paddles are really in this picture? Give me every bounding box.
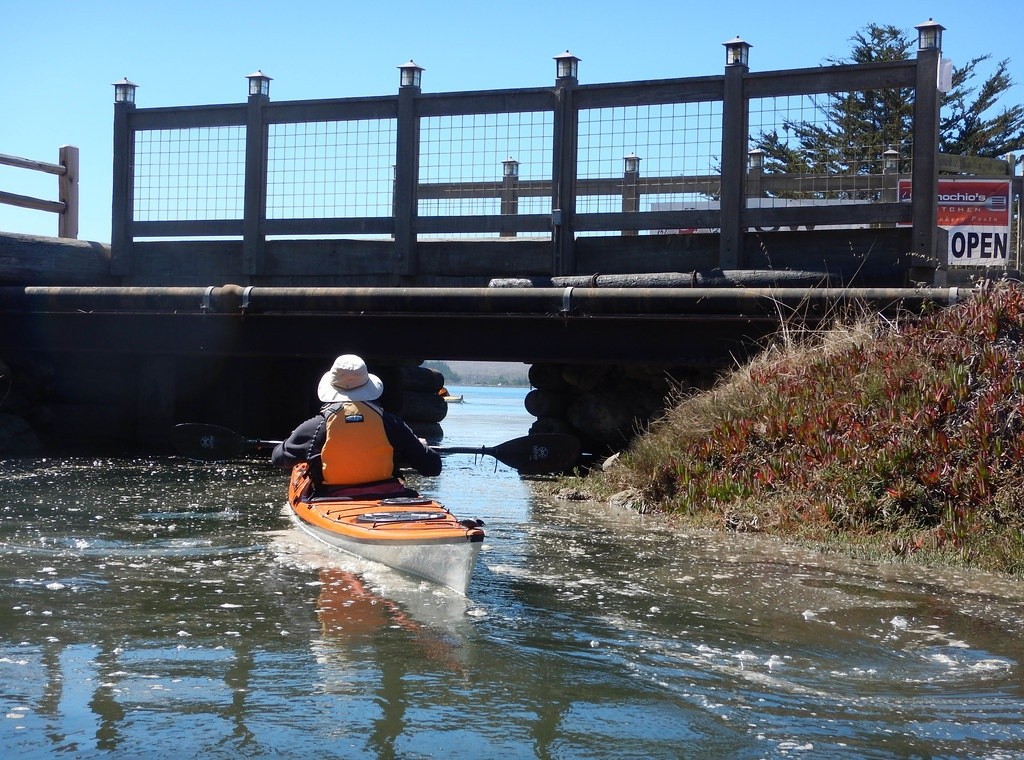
[167,423,580,474]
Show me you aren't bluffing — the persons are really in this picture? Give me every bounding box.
[271,355,442,495]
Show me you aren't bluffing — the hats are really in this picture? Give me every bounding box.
[317,354,384,402]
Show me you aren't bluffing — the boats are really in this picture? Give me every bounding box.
[288,462,484,596]
